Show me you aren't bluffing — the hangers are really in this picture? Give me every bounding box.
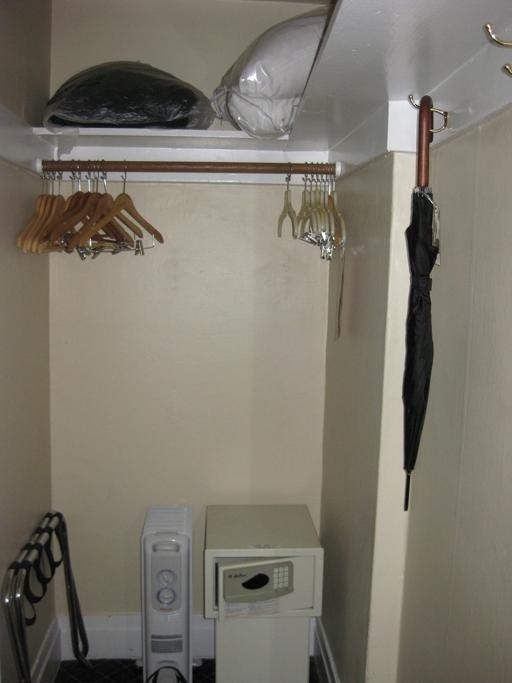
[279,161,348,260]
[16,155,163,257]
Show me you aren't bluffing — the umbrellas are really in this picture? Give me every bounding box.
[401,96,442,512]
